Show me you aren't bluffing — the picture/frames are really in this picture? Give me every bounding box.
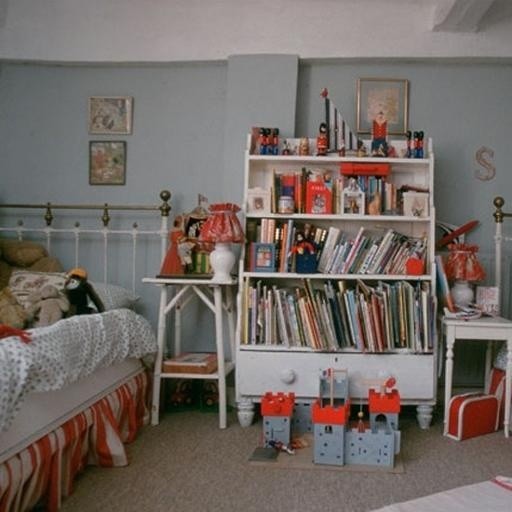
[250,240,277,273]
[340,188,366,217]
[87,95,134,136]
[245,187,271,216]
[402,190,429,220]
[356,77,409,136]
[88,140,127,186]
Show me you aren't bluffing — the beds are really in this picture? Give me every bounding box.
[493,194,510,441]
[1,190,173,511]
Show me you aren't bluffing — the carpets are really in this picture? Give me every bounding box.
[368,473,512,512]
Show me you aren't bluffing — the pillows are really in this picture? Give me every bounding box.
[4,267,70,315]
[86,278,141,315]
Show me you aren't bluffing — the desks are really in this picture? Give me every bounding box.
[437,314,512,439]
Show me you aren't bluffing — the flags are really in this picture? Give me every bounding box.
[198,192,209,206]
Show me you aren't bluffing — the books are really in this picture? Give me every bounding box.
[163,351,219,374]
[244,171,435,353]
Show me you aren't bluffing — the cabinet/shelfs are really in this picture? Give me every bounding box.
[235,132,439,430]
[142,277,237,431]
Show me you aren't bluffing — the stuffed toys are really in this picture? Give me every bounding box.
[62,267,106,317]
[28,284,68,327]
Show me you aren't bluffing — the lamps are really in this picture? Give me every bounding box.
[446,244,487,312]
[196,202,247,282]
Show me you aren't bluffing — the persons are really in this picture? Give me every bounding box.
[248,110,432,163]
[160,215,194,274]
